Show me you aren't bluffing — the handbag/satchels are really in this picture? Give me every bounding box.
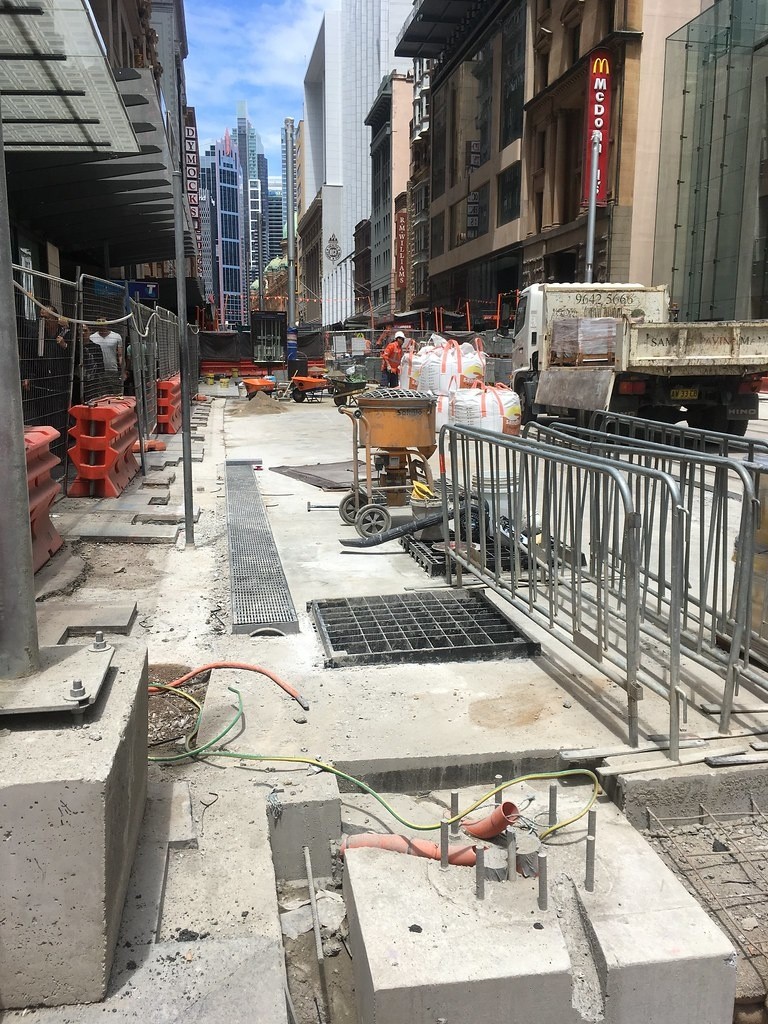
[399,337,521,441]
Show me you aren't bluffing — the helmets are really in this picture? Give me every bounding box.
[395,331,406,340]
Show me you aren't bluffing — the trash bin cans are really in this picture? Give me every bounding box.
[288,351,308,380]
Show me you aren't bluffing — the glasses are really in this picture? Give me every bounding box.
[97,319,109,323]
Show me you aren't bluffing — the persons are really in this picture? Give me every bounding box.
[380,330,406,388]
[18,306,148,464]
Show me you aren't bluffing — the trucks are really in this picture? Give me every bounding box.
[496,282,768,445]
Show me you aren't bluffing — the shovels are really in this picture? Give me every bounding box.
[277,370,298,402]
